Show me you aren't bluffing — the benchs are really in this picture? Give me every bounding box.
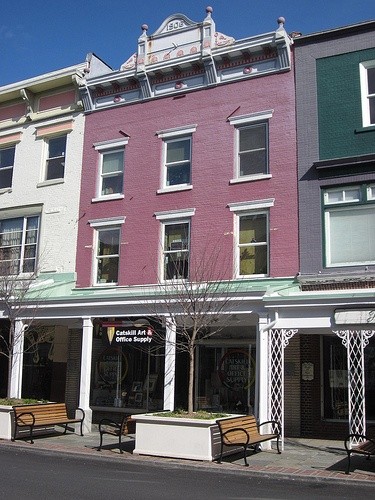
[11,401,86,444]
[97,408,170,454]
[215,415,285,466]
[344,429,375,474]
